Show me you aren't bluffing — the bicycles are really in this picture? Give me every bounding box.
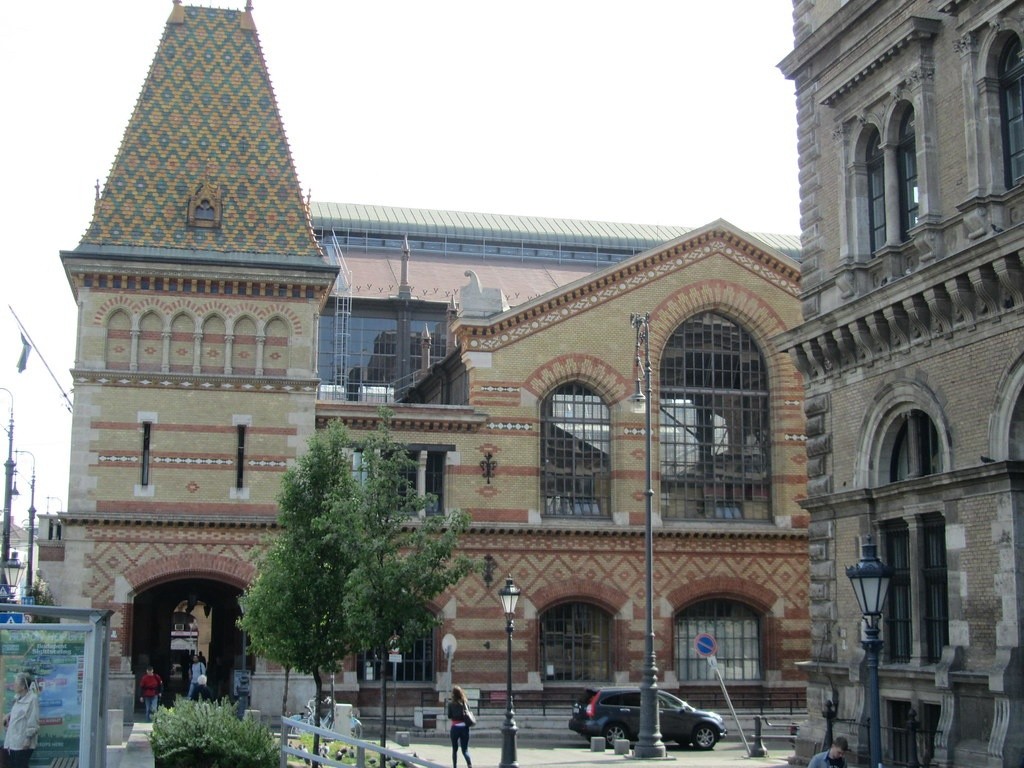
[289,695,363,741]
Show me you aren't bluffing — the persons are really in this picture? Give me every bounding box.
[806,736,850,768]
[196,651,207,670]
[2,671,42,768]
[187,655,206,698]
[191,674,214,706]
[138,665,164,723]
[447,685,475,767]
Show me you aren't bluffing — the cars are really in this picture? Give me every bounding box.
[787,720,808,750]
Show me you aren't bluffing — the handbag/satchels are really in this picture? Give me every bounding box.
[154,674,163,693]
[462,700,476,728]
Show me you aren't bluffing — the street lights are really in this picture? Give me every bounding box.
[843,531,894,767]
[237,590,253,721]
[11,449,39,620]
[496,573,522,768]
[629,310,670,759]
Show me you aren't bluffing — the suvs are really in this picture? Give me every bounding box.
[568,687,729,750]
